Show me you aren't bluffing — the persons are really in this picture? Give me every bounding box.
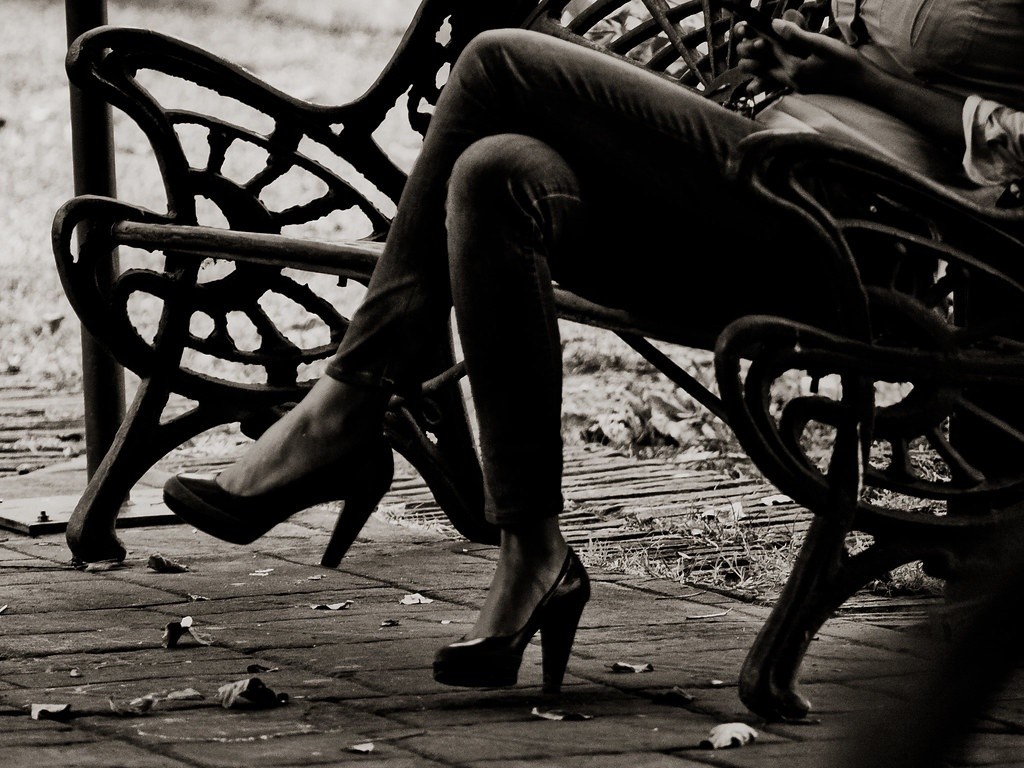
[162,0,1024,697]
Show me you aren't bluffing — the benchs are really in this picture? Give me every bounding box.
[52,0,1024,723]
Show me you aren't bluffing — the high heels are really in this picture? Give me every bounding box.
[432,545,590,693]
[163,434,394,569]
